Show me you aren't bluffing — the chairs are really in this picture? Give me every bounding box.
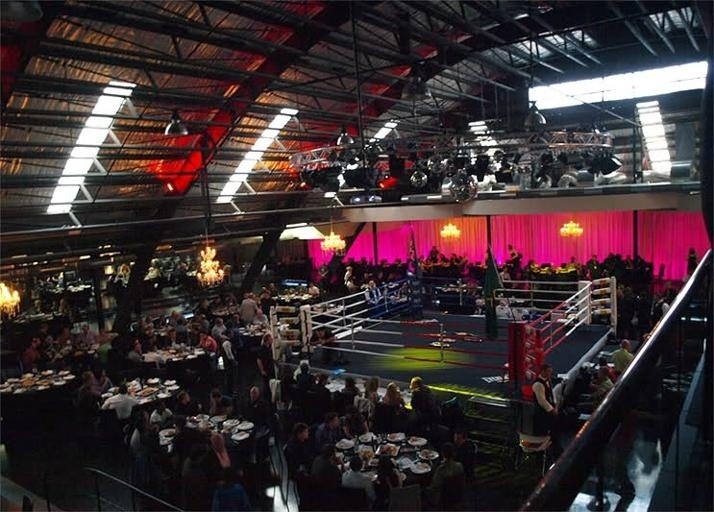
[516,338,637,480]
[324,251,676,312]
[325,393,479,511]
[0,253,324,510]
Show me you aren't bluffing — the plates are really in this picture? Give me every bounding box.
[100,377,180,398]
[155,345,204,359]
[334,431,439,477]
[0,369,74,394]
[191,414,255,441]
[238,324,267,337]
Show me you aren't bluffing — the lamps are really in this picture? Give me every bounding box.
[161,115,190,139]
[188,241,230,286]
[321,220,351,254]
[522,98,548,129]
[332,123,358,149]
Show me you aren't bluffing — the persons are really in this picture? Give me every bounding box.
[338,246,489,316]
[532,339,634,467]
[298,124,629,189]
[313,377,474,512]
[3,254,340,512]
[495,245,678,341]
